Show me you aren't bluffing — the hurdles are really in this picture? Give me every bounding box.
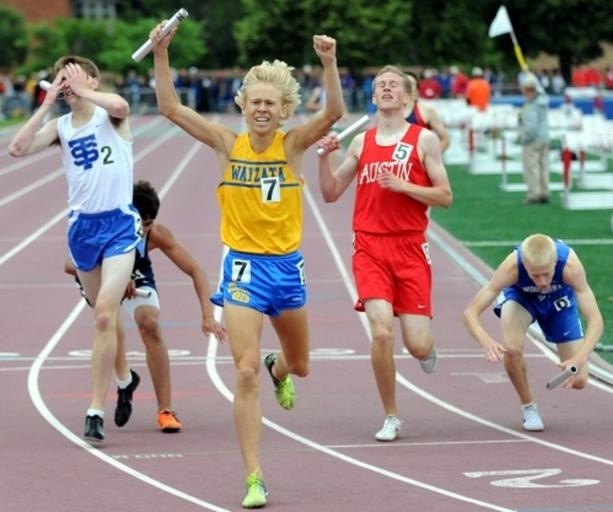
[433,99,613,211]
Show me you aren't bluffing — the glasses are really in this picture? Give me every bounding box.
[143,220,153,227]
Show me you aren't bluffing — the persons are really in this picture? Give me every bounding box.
[62,180,232,433]
[0,37,612,120]
[461,232,605,432]
[6,54,145,445]
[401,70,451,155]
[512,66,552,206]
[314,62,454,442]
[149,19,348,509]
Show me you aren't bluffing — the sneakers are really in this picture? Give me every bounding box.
[115,368,140,427]
[264,353,296,410]
[82,414,105,446]
[520,400,544,431]
[241,466,269,508]
[419,350,436,374]
[374,416,402,441]
[155,408,182,432]
